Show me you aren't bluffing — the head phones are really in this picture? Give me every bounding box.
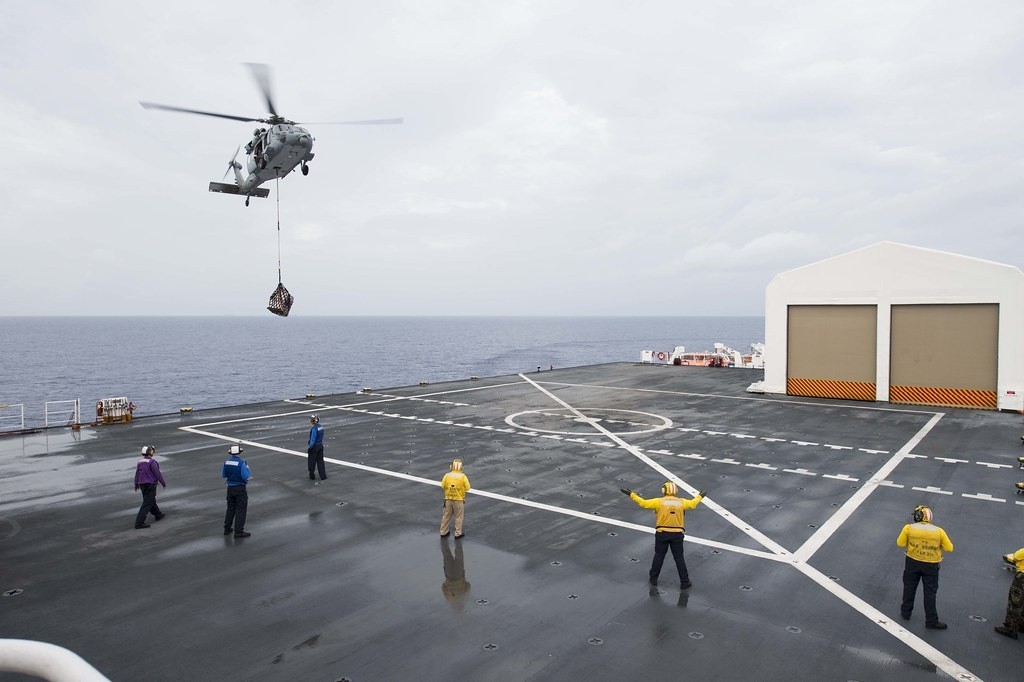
[662,488,678,495]
[314,414,317,423]
[228,448,243,455]
[450,464,464,470]
[148,445,153,455]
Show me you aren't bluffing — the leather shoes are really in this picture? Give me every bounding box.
[134,523,150,529]
[224,528,233,535]
[925,622,948,630]
[234,532,251,538]
[155,512,165,521]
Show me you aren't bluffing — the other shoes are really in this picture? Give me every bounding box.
[680,580,692,589]
[650,575,658,585]
[440,531,450,537]
[454,532,465,538]
[995,626,1018,639]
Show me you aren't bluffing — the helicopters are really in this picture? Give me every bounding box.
[134,63,404,207]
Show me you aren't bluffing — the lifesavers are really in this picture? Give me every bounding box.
[658,352,665,360]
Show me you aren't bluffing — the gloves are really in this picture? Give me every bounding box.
[699,489,707,498]
[620,488,633,496]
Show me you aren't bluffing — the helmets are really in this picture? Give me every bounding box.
[449,459,464,472]
[227,443,244,455]
[141,445,156,457]
[911,504,933,522]
[310,413,319,423]
[661,481,679,495]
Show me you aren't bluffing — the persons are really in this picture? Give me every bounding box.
[995,546,1024,640]
[221,443,252,538]
[439,459,471,539]
[620,481,706,589]
[133,444,166,529]
[897,504,953,629]
[306,414,327,480]
